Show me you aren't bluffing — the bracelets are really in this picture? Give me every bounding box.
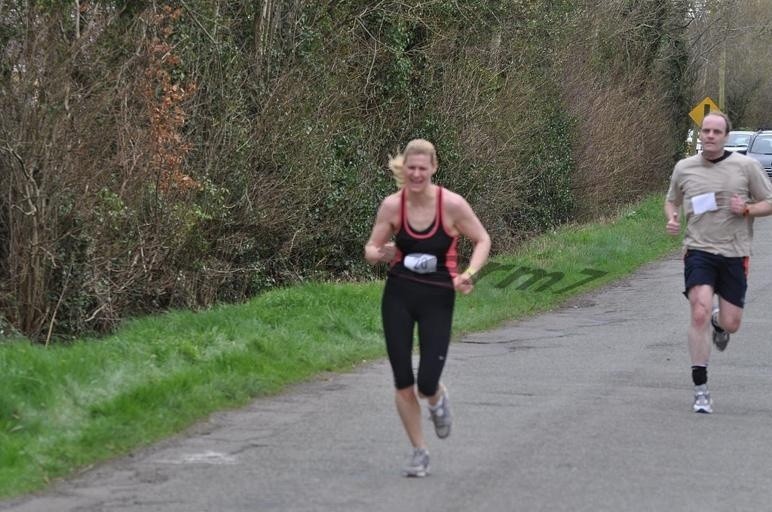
[465,267,478,283]
[742,207,749,217]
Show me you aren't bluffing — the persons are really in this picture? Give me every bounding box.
[363,138,493,479]
[662,111,772,414]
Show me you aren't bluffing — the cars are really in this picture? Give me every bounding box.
[745,130,771,179]
[683,129,757,156]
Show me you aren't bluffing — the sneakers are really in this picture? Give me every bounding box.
[711,309,729,351]
[428,384,454,439]
[693,384,713,414]
[403,449,431,477]
